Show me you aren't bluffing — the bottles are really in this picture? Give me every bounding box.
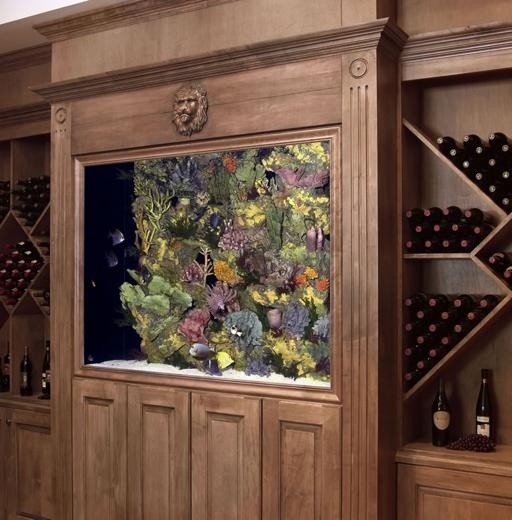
[0,178,52,316]
[434,132,511,210]
[430,375,453,446]
[488,251,512,282]
[403,291,502,387]
[3,341,9,389]
[18,346,30,396]
[475,367,494,442]
[404,206,497,253]
[39,339,51,395]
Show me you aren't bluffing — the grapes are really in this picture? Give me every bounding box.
[447,432,495,452]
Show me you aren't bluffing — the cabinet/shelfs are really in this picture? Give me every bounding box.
[0,125,73,520]
[394,46,512,520]
